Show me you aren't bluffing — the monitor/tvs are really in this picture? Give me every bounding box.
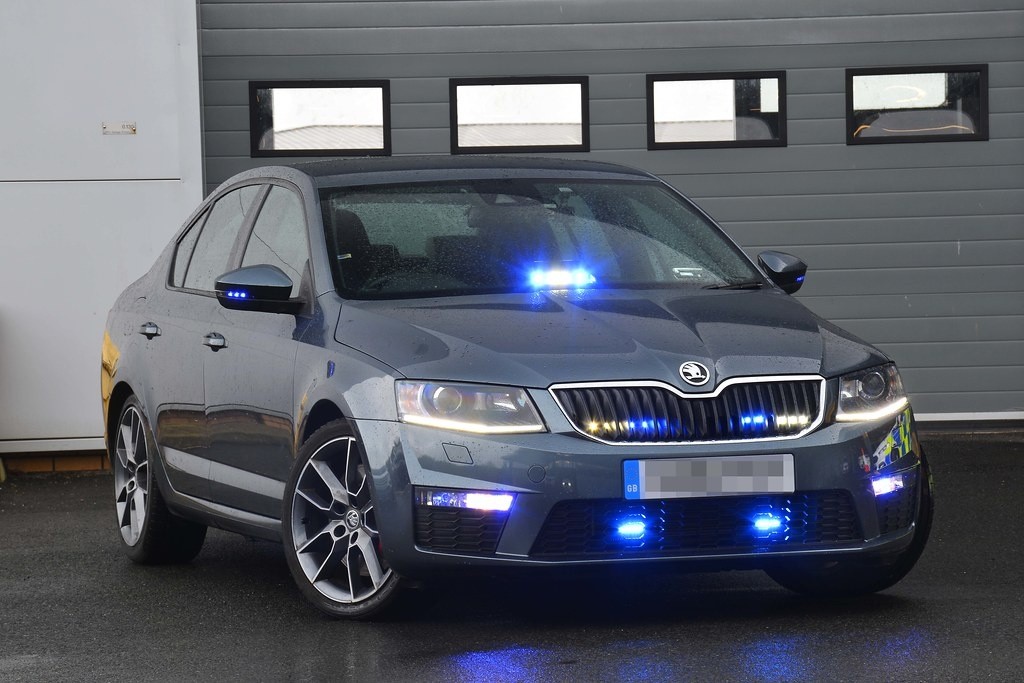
[248,80,392,156]
[448,78,591,154]
[845,64,990,146]
[646,70,788,150]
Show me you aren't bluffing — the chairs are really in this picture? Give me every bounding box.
[330,210,400,272]
[430,234,493,275]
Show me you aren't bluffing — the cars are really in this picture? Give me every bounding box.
[97,151,936,627]
